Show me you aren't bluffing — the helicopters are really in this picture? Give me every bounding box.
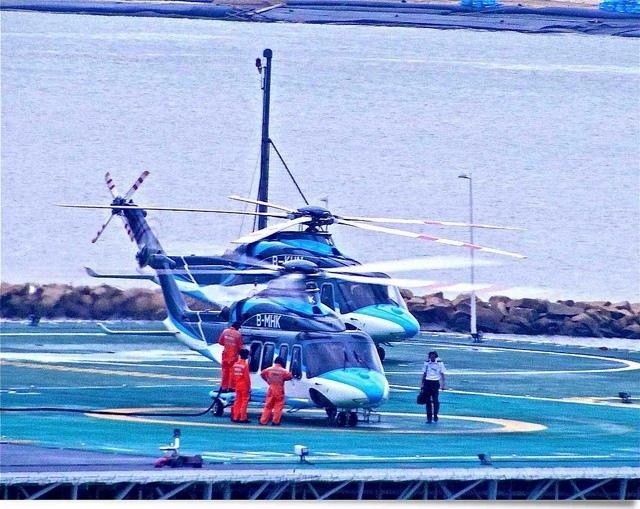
[82,246,494,430]
[52,165,535,360]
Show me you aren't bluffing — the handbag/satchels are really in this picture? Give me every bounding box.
[417,390,424,404]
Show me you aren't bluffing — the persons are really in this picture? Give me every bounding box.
[219,319,244,392]
[258,357,293,425]
[231,349,252,423]
[420,350,446,424]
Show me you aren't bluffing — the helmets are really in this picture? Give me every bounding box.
[429,350,438,357]
